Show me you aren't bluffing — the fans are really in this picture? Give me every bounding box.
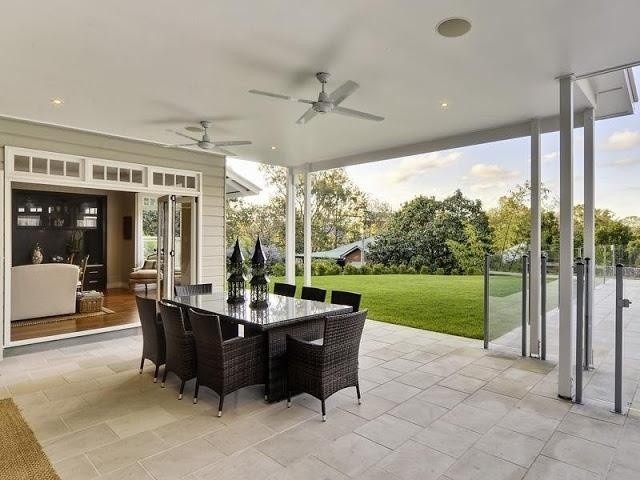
[162,118,254,158]
[246,70,384,127]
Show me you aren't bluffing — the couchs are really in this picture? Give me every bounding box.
[12,261,80,323]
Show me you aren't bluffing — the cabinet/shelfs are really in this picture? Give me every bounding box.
[83,265,105,292]
[12,190,101,231]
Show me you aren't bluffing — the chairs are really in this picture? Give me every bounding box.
[76,253,93,292]
[135,280,373,425]
[67,251,75,264]
[126,251,165,297]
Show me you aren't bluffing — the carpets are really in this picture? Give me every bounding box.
[0,394,62,480]
[10,305,116,329]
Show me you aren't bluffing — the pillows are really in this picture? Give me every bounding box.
[141,259,164,271]
